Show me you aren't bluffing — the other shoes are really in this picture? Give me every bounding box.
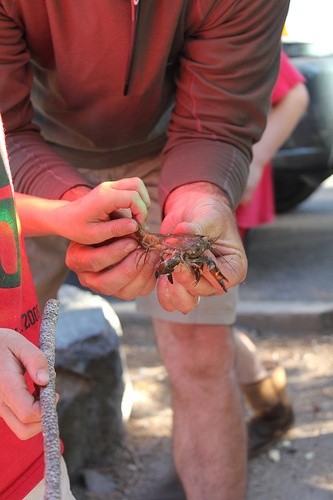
[247,402,294,458]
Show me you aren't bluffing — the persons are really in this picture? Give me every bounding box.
[0,0,291,500]
[229,47,309,460]
[0,113,150,500]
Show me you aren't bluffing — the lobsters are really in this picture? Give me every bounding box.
[131,219,242,294]
